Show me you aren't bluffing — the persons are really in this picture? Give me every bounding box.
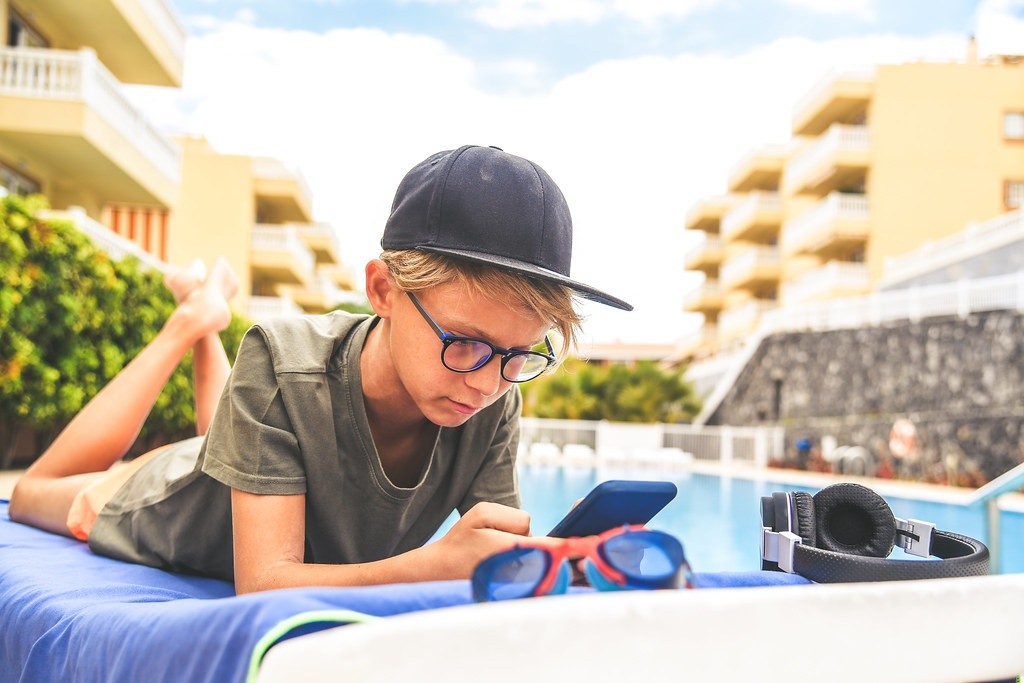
[6,140,646,599]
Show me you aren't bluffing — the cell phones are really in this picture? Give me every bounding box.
[545,480,677,538]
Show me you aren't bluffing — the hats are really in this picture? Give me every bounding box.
[380,144,634,312]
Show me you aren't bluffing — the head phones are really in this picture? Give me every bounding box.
[760,483,991,584]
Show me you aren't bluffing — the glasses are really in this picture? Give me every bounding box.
[471,523,686,602]
[404,289,557,383]
